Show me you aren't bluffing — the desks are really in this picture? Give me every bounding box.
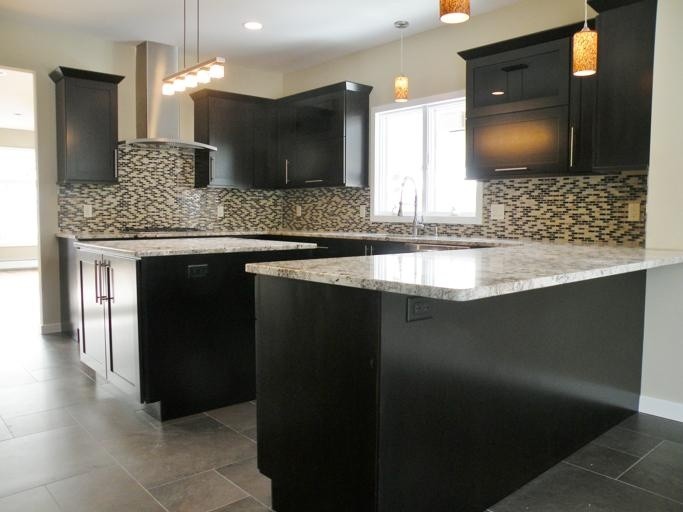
[246,237,648,511]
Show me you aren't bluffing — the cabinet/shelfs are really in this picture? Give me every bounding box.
[316,238,488,258]
[296,82,374,190]
[464,107,570,182]
[454,16,597,112]
[49,64,125,186]
[188,88,277,190]
[579,1,658,178]
[59,240,317,424]
[277,95,296,189]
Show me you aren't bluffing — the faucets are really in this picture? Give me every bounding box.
[397,175,419,225]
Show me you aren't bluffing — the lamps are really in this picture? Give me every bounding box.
[161,1,226,98]
[574,1,598,78]
[393,20,409,105]
[439,1,472,24]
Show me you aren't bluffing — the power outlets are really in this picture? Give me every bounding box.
[187,263,209,280]
[405,297,438,323]
